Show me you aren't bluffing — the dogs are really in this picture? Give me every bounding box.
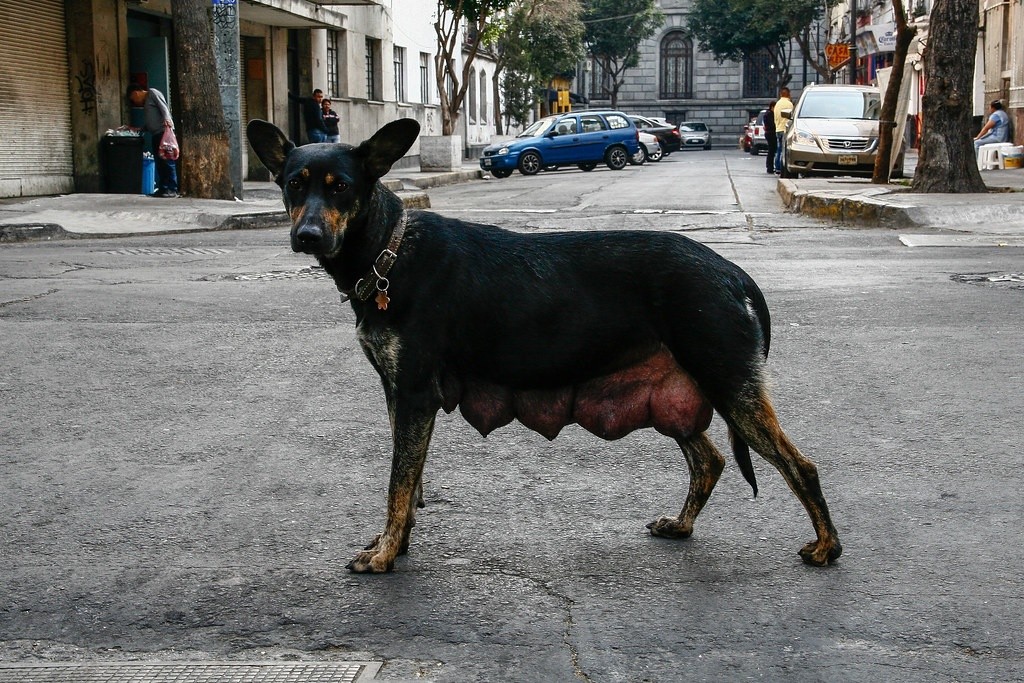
[244,116,842,576]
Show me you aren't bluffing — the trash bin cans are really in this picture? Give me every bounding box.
[143,157,156,194]
[96,135,143,194]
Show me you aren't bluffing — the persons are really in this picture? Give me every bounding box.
[973,100,1011,159]
[303,89,343,144]
[765,87,795,174]
[125,83,181,198]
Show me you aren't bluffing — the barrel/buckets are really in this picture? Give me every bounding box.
[1001,146,1023,169]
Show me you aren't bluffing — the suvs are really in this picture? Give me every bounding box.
[479,109,639,179]
[780,80,906,179]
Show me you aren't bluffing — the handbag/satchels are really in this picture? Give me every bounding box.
[159,126,179,160]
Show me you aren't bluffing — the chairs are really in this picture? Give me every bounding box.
[571,124,577,134]
[559,125,568,135]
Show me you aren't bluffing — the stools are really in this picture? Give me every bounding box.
[978,143,1014,171]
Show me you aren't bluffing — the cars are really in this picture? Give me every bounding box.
[741,110,767,155]
[679,121,713,150]
[542,115,680,172]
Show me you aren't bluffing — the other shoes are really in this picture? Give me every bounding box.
[151,187,176,198]
[146,189,159,197]
[767,168,776,174]
